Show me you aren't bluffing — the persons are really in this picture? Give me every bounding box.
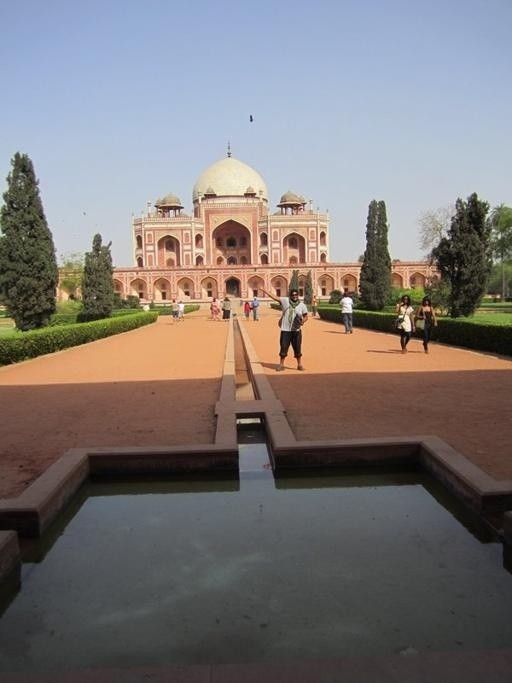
[310,294,320,317]
[338,292,355,334]
[244,302,252,321]
[250,296,260,321]
[209,297,221,321]
[143,302,150,311]
[178,300,185,321]
[394,293,416,354]
[259,283,308,372]
[415,296,438,353]
[221,297,232,322]
[172,299,179,321]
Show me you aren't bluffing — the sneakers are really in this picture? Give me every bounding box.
[297,366,303,370]
[276,366,284,371]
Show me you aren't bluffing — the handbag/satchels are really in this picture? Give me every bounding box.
[391,318,403,331]
[277,318,281,328]
[415,319,425,330]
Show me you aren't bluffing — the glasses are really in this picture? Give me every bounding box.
[292,294,297,297]
